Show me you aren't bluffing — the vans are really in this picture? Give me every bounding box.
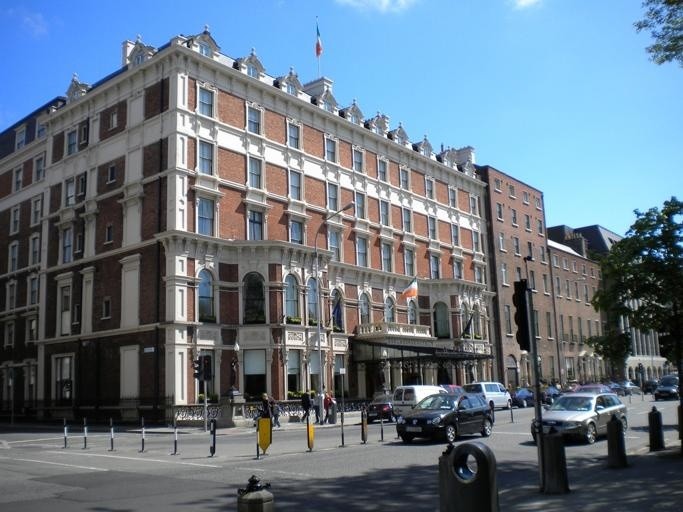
[392,382,512,418]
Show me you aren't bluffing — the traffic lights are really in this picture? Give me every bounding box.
[512,281,530,351]
[192,356,211,381]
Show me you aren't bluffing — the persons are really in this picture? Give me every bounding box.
[261,390,333,428]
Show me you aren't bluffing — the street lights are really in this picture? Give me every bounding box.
[314,201,355,425]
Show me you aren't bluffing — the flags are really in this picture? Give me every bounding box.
[332,299,342,330]
[400,278,417,300]
[464,317,473,335]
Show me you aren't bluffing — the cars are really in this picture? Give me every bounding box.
[366,395,399,423]
[396,393,494,442]
[531,393,627,444]
[642,375,680,400]
[512,381,642,408]
